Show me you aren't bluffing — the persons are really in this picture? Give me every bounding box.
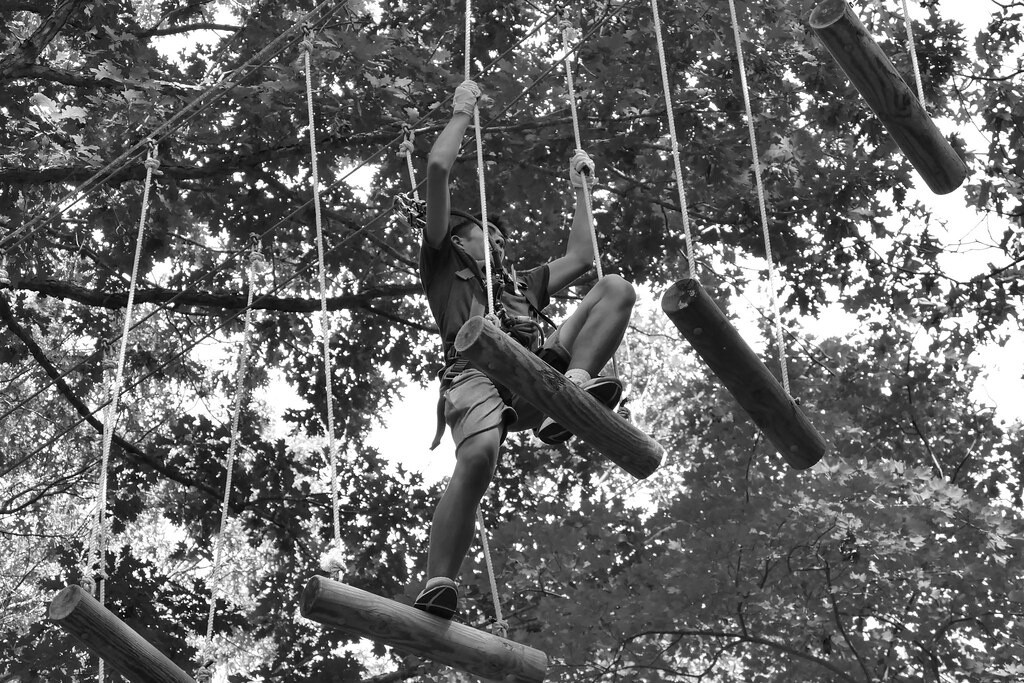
[412,79,636,620]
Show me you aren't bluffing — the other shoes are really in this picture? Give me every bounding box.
[414,579,460,620]
[538,377,623,445]
